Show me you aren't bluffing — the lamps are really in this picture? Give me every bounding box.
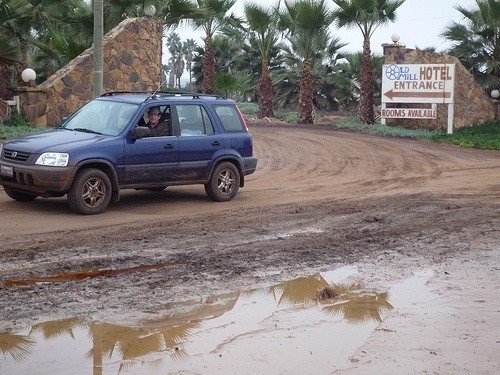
[21,68,37,88]
[144,3,156,19]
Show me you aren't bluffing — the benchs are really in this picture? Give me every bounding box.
[179,115,240,134]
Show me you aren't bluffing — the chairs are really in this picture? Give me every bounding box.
[160,113,172,122]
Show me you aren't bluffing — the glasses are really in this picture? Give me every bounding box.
[148,112,160,117]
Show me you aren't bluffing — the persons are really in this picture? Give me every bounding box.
[143,105,170,137]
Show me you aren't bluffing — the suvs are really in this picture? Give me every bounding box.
[0,90,257,216]
